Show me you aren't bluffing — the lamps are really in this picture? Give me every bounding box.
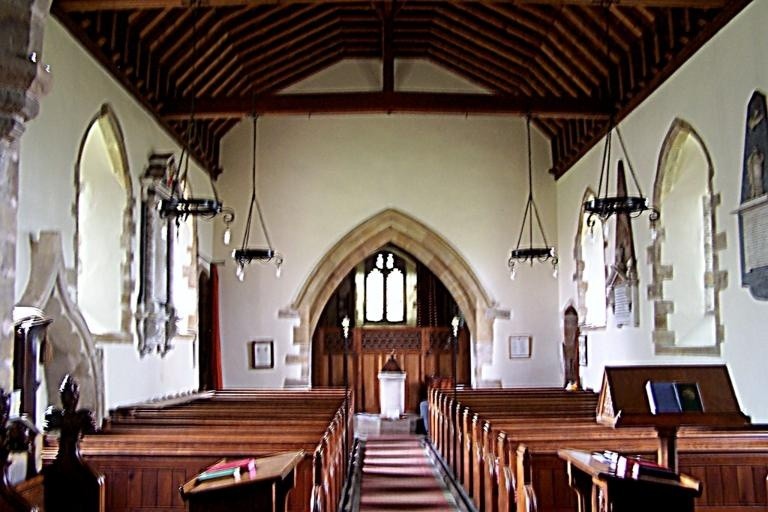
[157,0,234,246]
[584,0,660,241]
[232,117,284,282]
[506,120,559,280]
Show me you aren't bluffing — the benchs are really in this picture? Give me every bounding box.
[428,386,768,512]
[41,388,354,512]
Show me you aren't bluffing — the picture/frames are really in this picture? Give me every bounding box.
[508,336,532,359]
[251,339,274,370]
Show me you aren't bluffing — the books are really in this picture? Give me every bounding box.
[196,467,241,483]
[207,457,256,472]
[646,380,680,416]
[590,448,678,480]
[671,380,706,412]
